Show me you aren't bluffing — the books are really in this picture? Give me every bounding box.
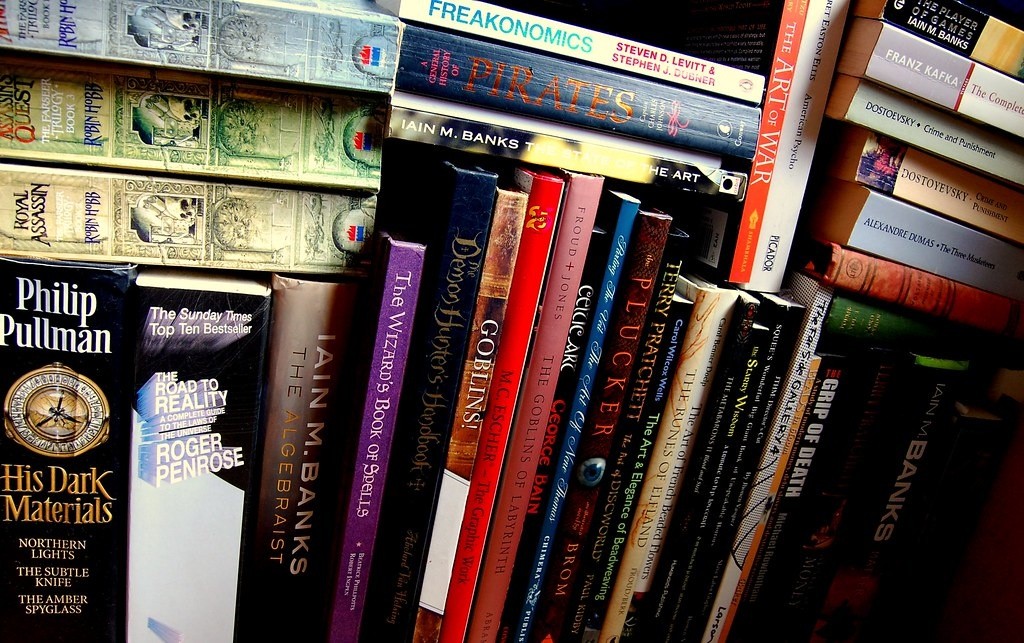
[0,0,766,273]
[0,171,1024,643]
[689,0,1024,331]
